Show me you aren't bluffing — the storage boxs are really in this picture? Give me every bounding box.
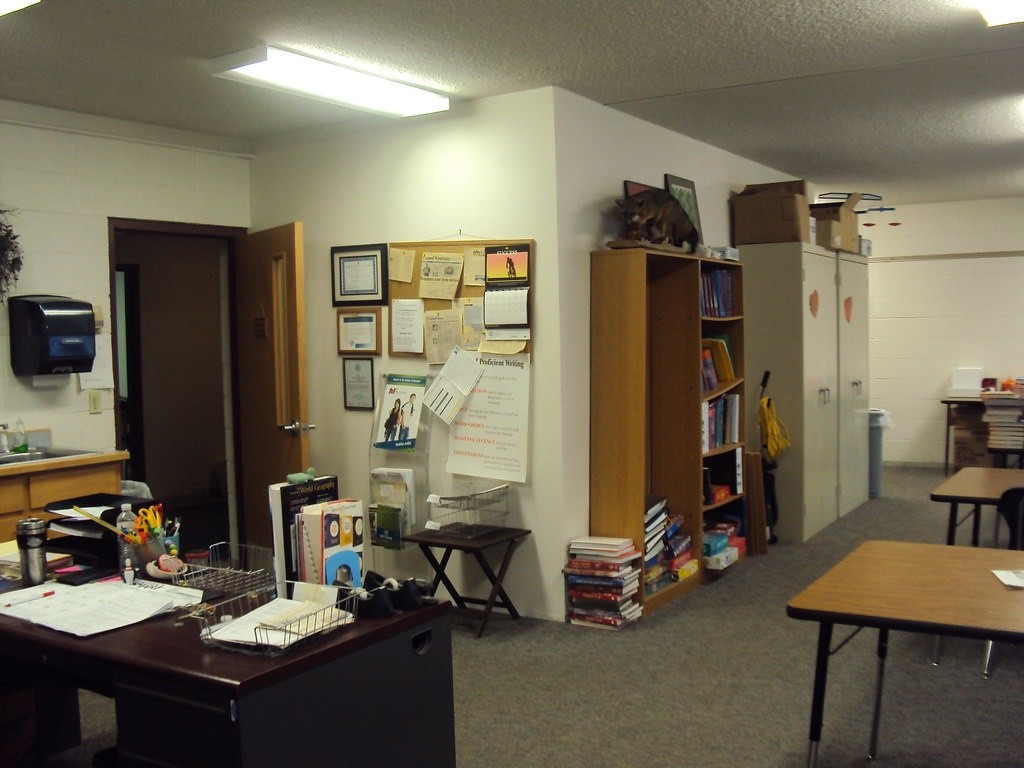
[711,180,872,262]
[700,521,747,570]
[948,367,983,398]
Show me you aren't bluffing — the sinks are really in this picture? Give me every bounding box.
[0,451,104,466]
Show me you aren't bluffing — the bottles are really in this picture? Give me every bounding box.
[124,558,134,585]
[13,418,28,453]
[117,503,137,568]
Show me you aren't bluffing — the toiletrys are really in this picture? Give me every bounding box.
[12,417,28,452]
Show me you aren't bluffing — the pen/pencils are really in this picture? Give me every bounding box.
[4,590,56,606]
[118,503,181,547]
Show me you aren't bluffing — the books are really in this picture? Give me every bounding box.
[560,536,643,632]
[368,467,416,550]
[981,376,1024,449]
[266,474,364,599]
[644,268,747,594]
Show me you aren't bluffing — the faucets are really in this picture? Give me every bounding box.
[0,421,9,432]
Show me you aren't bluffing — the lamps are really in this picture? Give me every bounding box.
[206,44,450,121]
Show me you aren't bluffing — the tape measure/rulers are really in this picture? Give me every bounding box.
[72,504,128,539]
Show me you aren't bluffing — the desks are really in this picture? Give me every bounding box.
[402,522,532,639]
[0,560,455,768]
[931,467,1024,547]
[940,398,1024,479]
[786,539,1024,768]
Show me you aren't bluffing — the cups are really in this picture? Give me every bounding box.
[136,532,166,565]
[162,532,179,557]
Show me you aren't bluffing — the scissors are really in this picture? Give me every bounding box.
[139,505,168,554]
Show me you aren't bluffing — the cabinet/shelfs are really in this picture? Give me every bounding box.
[734,241,869,545]
[590,248,768,622]
[0,461,121,552]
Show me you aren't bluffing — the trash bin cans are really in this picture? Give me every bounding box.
[868,408,885,500]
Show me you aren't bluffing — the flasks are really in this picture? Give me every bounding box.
[16,517,47,586]
